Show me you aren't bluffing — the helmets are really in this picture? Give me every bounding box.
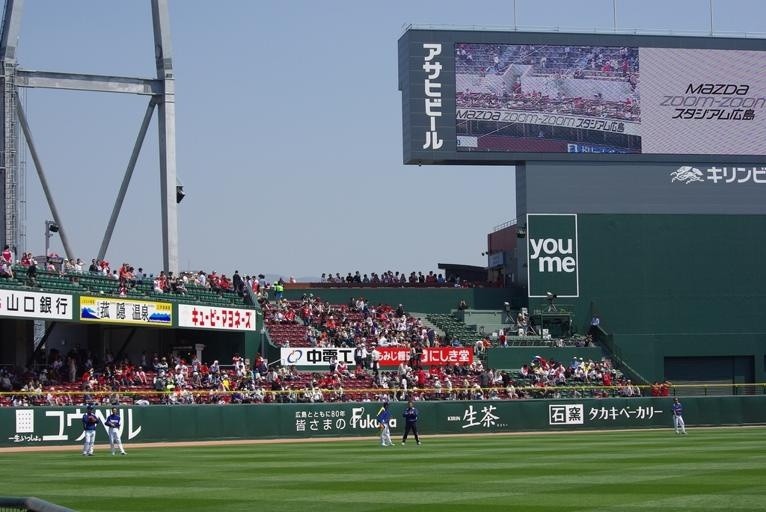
[87,405,94,410]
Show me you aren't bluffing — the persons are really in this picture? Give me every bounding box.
[456,43,641,146]
[671,397,686,434]
[401,401,421,445]
[82,405,99,456]
[105,407,128,456]
[376,402,395,447]
[1,246,674,407]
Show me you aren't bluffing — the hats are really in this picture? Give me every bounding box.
[518,313,522,318]
[138,270,482,403]
[23,252,129,266]
[40,368,49,373]
[476,341,483,347]
[485,355,632,397]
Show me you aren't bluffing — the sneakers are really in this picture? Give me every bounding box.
[121,452,128,455]
[401,442,406,446]
[381,443,388,447]
[416,441,422,446]
[83,453,95,457]
[388,442,395,446]
[112,452,116,456]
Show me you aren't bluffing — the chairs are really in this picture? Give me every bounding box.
[0,262,643,409]
[453,42,641,124]
